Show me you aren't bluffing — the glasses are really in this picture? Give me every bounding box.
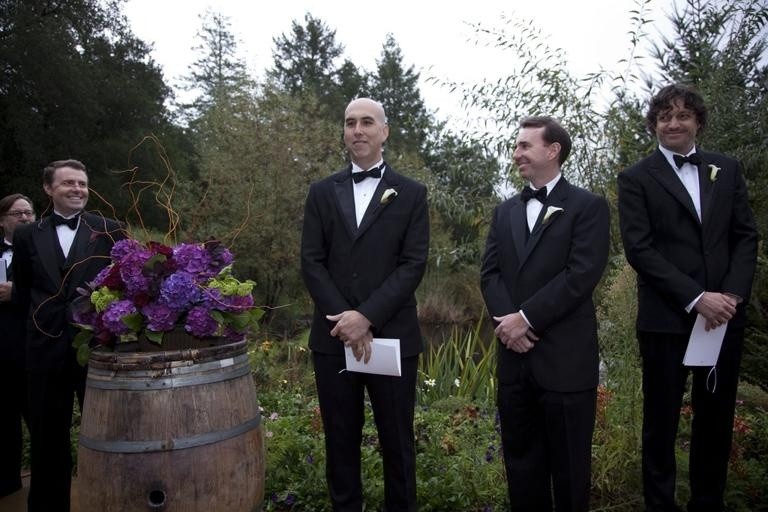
[3,210,33,218]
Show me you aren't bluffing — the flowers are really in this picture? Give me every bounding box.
[32,131,292,368]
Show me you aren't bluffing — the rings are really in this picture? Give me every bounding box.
[718,317,725,323]
[344,339,351,346]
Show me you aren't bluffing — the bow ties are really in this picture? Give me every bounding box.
[0,242,13,252]
[52,214,77,230]
[352,167,380,183]
[672,153,701,169]
[519,186,546,204]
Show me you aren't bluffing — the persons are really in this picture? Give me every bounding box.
[11,158,130,510]
[478,115,611,511]
[617,83,760,511]
[299,97,431,512]
[1,193,39,498]
